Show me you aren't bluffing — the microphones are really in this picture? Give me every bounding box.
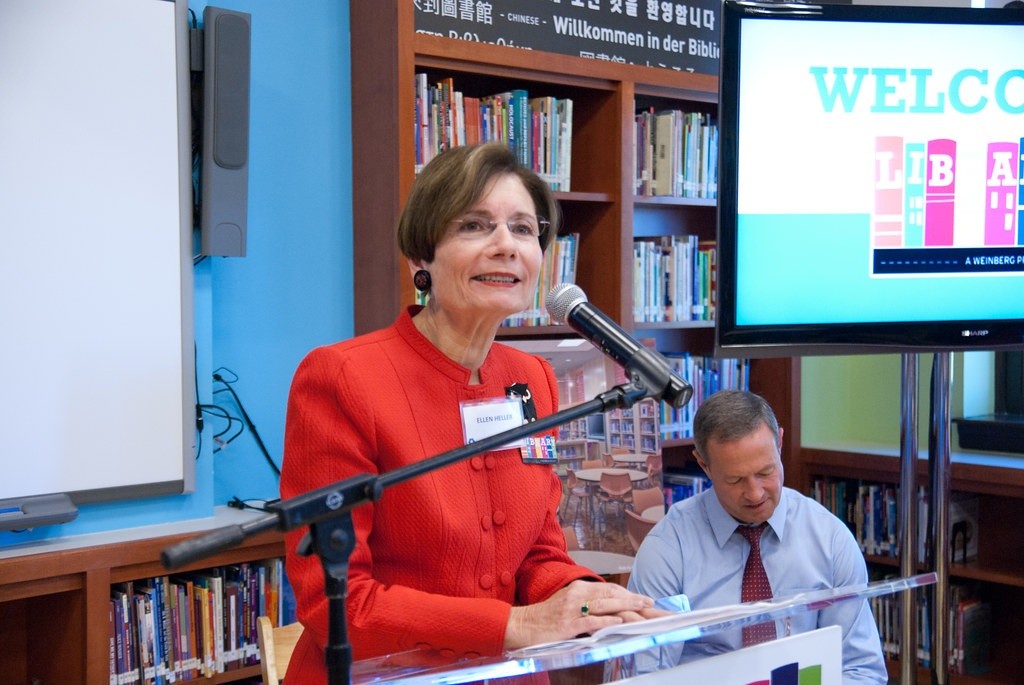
[546,282,693,410]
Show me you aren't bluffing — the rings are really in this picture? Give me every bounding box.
[581,600,589,616]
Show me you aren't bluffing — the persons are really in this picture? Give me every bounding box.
[602,389,892,685]
[280,135,674,685]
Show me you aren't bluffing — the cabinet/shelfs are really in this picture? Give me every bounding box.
[803,436,1023,684]
[0,500,286,684]
[352,1,803,495]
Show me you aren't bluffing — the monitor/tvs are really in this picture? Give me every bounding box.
[713,0,1024,359]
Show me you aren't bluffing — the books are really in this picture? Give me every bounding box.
[556,403,654,473]
[663,471,711,517]
[807,474,990,675]
[112,557,283,685]
[414,69,753,447]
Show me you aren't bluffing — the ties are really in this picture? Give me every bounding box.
[733,521,778,649]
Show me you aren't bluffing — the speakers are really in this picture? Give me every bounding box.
[197,6,252,258]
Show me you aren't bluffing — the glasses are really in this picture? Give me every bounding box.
[445,213,551,238]
